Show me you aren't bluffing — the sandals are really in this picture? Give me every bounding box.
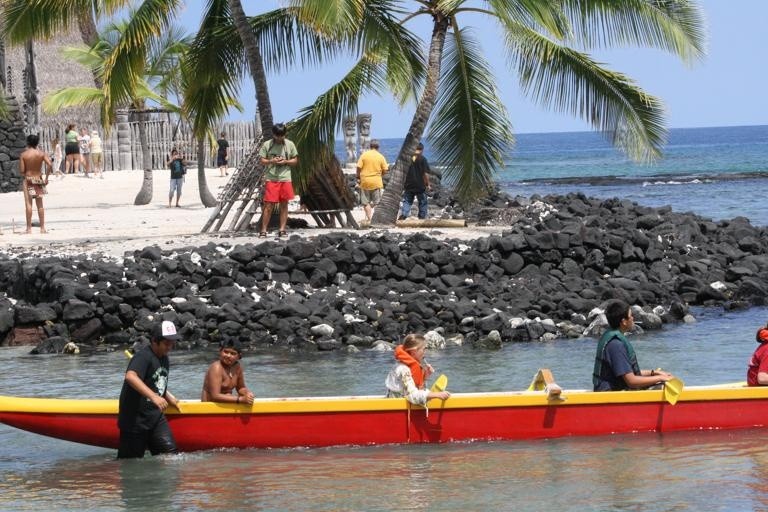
[260,231,288,238]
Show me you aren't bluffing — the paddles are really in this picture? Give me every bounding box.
[430,375,448,392]
[658,367,683,405]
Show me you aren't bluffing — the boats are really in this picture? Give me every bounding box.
[0,381,768,451]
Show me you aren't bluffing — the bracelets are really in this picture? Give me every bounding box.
[651,369,655,375]
[237,397,240,404]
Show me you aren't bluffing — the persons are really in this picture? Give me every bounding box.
[593,302,672,391]
[386,333,451,405]
[217,132,229,176]
[117,321,180,459]
[399,142,430,219]
[20,135,51,233]
[167,151,187,207]
[201,339,255,405]
[51,124,104,179]
[258,123,299,238]
[356,140,388,221]
[747,325,768,387]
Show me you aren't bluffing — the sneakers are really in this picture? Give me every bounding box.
[398,215,407,220]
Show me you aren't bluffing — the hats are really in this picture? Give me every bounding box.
[150,321,182,340]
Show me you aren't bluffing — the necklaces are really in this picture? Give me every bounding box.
[225,365,233,379]
